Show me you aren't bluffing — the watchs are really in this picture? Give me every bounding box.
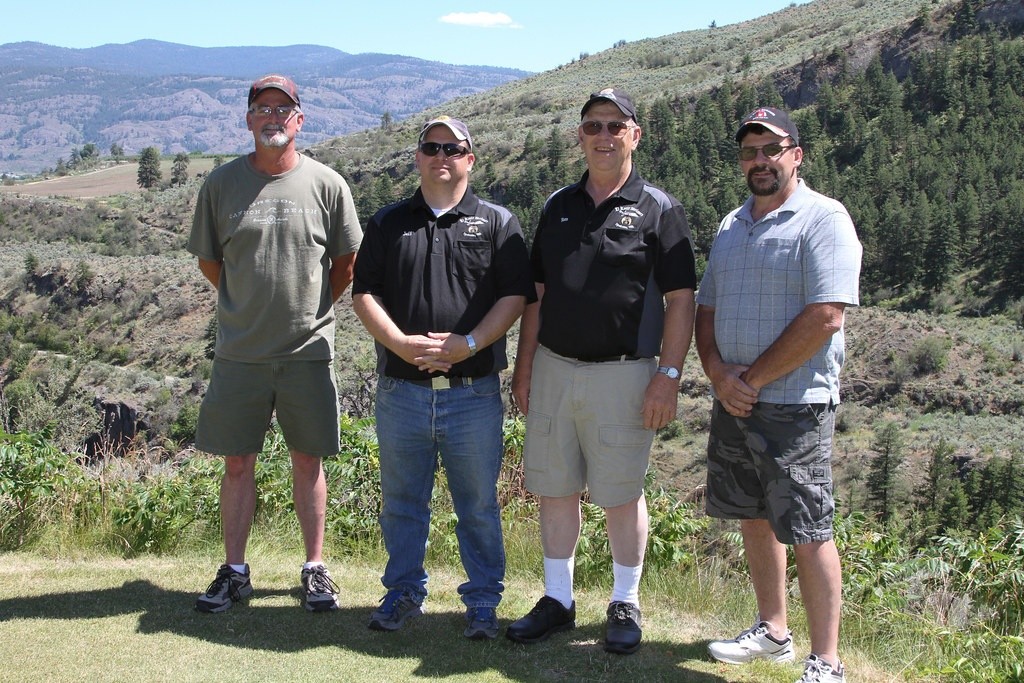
[657,366,683,381]
[465,335,476,357]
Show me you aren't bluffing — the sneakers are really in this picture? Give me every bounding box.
[795,653,847,683]
[462,607,500,639]
[194,563,253,613]
[706,620,795,665]
[300,565,340,612]
[367,584,426,629]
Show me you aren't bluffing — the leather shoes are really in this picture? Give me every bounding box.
[505,596,575,643]
[605,601,642,654]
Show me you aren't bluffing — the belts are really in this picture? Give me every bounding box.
[405,376,477,390]
[552,347,640,361]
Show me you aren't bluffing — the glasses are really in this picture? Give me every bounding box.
[739,143,794,161]
[249,104,299,119]
[419,142,470,159]
[581,120,635,136]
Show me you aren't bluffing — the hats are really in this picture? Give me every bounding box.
[580,88,637,124]
[248,74,301,109]
[735,106,799,147]
[418,115,472,152]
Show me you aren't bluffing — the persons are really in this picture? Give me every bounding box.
[186,73,363,615]
[691,106,864,683]
[351,116,540,639]
[506,87,696,654]
